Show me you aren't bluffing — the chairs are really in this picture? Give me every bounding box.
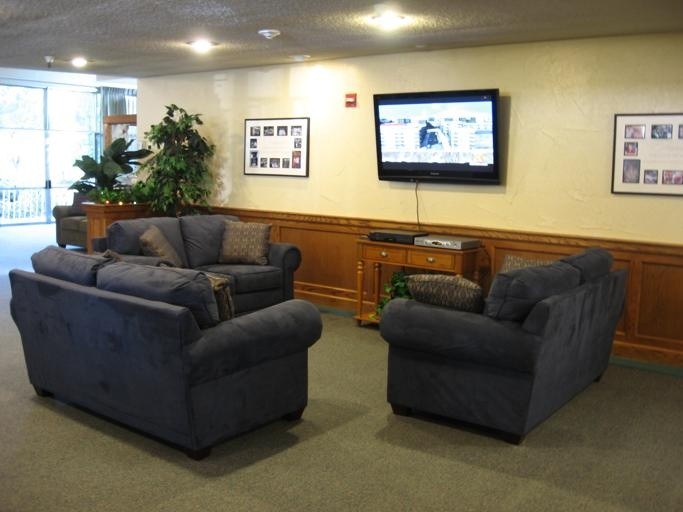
[51,193,89,250]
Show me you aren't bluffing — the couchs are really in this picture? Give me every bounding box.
[377,242,627,445]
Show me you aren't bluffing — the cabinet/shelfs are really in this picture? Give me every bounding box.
[351,239,481,326]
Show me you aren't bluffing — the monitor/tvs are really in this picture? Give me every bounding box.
[373,87,500,185]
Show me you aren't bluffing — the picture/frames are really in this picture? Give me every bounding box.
[608,110,683,197]
[242,117,312,178]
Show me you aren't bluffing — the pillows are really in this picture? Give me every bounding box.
[403,272,482,314]
[502,255,555,274]
